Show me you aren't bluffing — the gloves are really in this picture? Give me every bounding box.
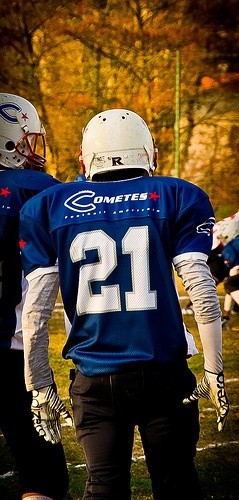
[182,370,230,432]
[31,385,73,445]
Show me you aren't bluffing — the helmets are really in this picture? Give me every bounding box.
[81,109,156,181]
[0,92,47,171]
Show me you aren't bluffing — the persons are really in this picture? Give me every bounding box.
[14,106,229,500]
[216,211,238,321]
[0,79,66,500]
[179,218,235,330]
